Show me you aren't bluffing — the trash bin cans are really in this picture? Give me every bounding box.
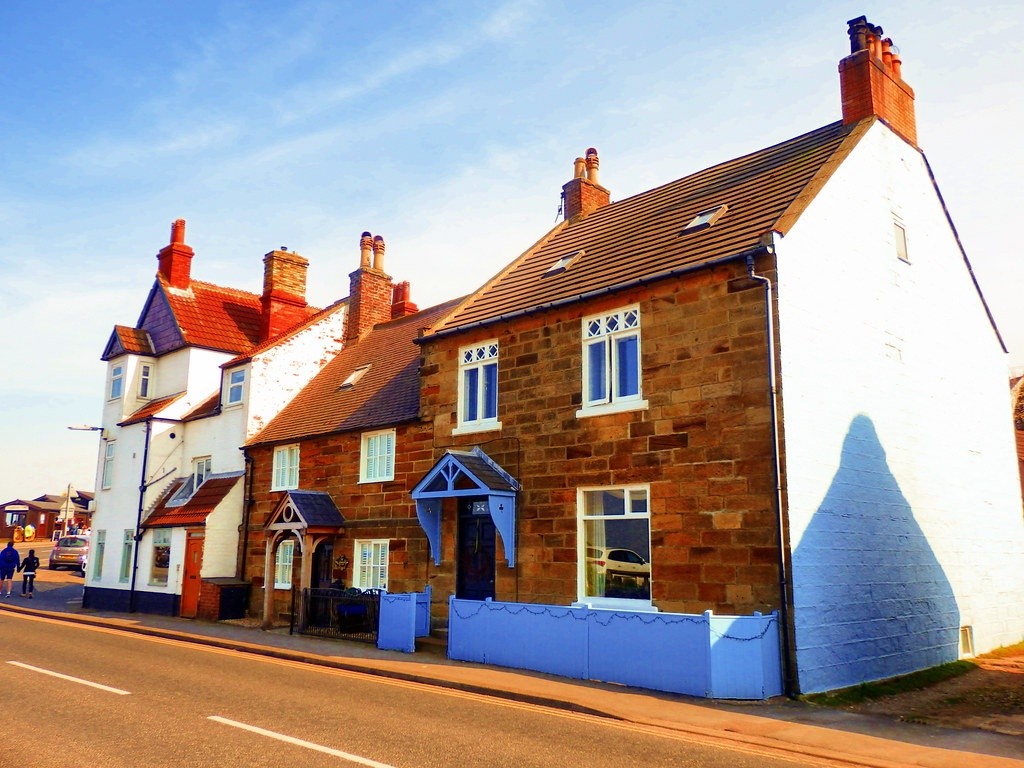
[24,524,37,541]
[336,603,365,637]
[13,526,24,542]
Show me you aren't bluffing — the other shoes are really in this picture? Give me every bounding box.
[19,593,27,598]
[26,592,32,599]
[5,594,11,598]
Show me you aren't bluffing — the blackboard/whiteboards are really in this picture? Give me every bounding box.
[52,530,61,541]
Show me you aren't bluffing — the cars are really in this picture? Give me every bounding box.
[49,534,88,573]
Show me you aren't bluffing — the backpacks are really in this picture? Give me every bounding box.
[3,548,15,564]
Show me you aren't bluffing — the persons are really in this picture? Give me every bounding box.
[17,550,40,599]
[0,541,20,598]
[68,520,91,535]
